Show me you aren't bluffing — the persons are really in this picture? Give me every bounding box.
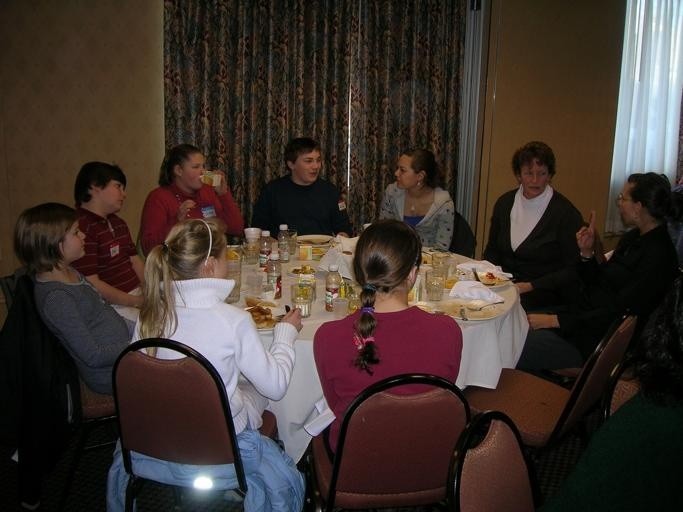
[14,203,130,395]
[516,172,678,380]
[141,144,244,259]
[74,161,146,310]
[252,137,352,238]
[314,218,464,464]
[379,148,454,252]
[484,141,583,295]
[129,220,304,444]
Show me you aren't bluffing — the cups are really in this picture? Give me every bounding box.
[224,272,242,303]
[425,270,444,302]
[227,260,241,287]
[241,238,260,265]
[289,230,297,256]
[432,252,450,271]
[291,284,313,318]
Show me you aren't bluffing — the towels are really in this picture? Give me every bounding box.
[457,259,513,279]
[450,280,503,303]
[320,250,352,281]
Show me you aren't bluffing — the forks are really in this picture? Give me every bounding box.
[466,301,504,311]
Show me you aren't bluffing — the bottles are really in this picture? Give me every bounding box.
[278,224,290,263]
[259,231,272,268]
[267,254,282,300]
[325,264,341,313]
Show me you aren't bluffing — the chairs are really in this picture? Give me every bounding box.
[461,309,639,506]
[16,274,118,511]
[535,365,584,391]
[111,338,279,512]
[445,411,543,512]
[307,373,472,512]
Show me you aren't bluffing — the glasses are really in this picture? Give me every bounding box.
[616,192,630,203]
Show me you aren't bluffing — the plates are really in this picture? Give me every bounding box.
[243,303,293,331]
[432,296,505,320]
[296,235,336,255]
[288,266,317,278]
[443,272,513,290]
